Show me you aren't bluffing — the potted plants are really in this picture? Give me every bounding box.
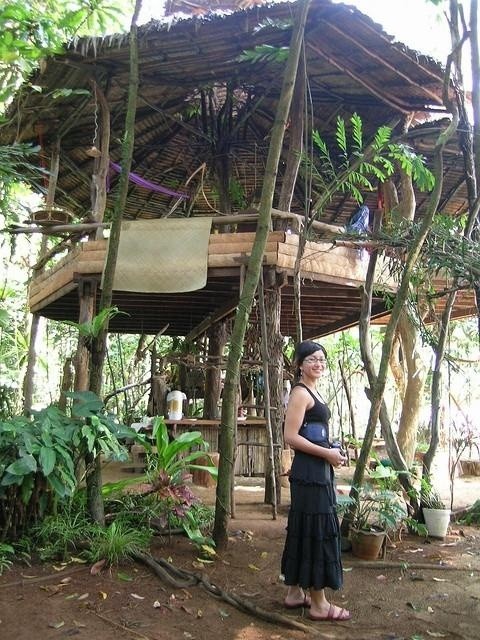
[329,432,454,562]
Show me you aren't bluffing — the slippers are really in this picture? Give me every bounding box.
[309,602,352,621]
[284,594,312,609]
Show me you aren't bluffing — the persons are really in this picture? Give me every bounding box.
[281,341,352,621]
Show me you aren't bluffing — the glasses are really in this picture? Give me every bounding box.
[303,359,327,363]
